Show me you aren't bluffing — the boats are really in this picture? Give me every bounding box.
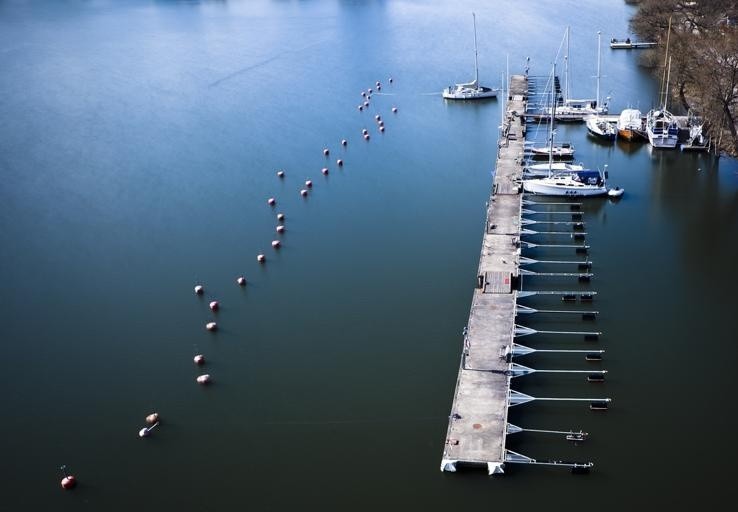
[616,108,643,141]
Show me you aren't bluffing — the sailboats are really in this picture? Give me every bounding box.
[443,10,500,102]
[647,16,682,151]
[523,21,617,198]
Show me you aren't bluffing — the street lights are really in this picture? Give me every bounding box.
[484,200,489,234]
[461,326,468,367]
